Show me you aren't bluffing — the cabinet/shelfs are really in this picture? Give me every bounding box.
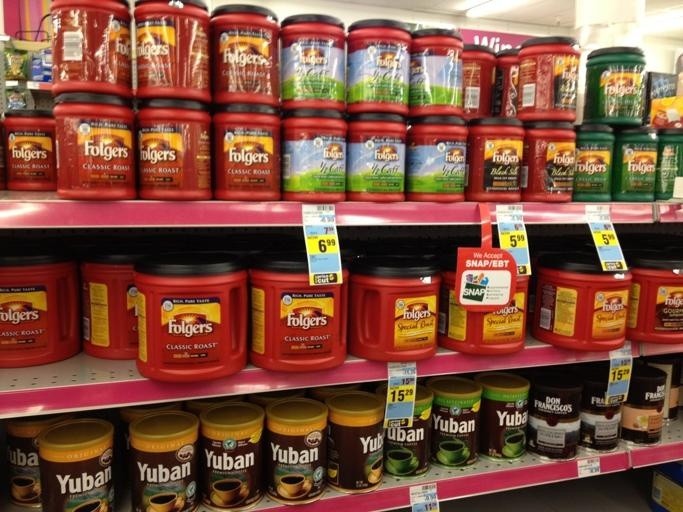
[0,190,682,512]
